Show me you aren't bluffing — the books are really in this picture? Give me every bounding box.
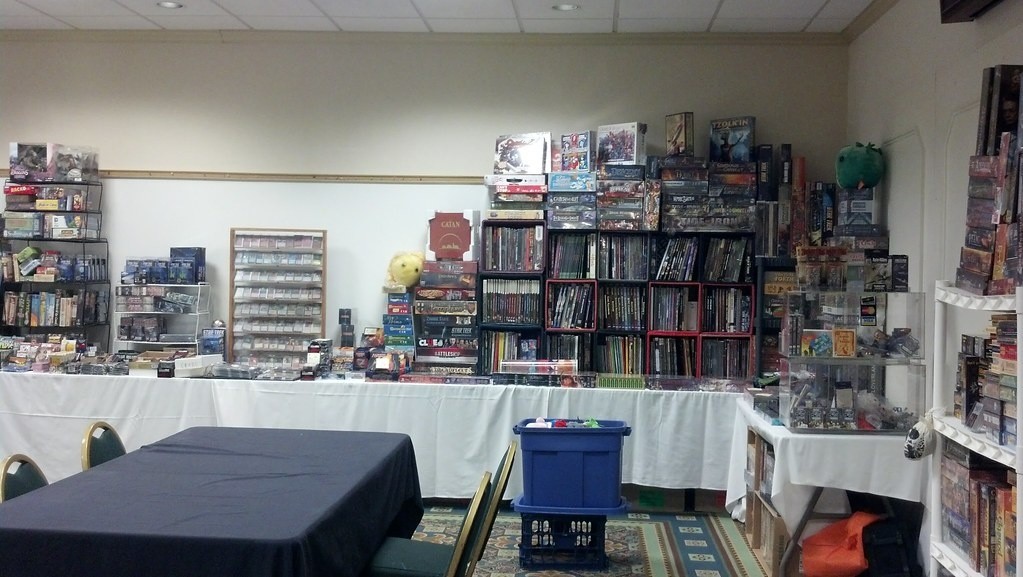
[158,300,194,315]
[116,295,160,305]
[954,64,1023,296]
[1,288,108,328]
[1,247,66,283]
[161,291,205,306]
[952,311,1017,451]
[383,111,890,380]
[939,434,1017,577]
[1,185,101,239]
[116,304,161,312]
[115,286,171,297]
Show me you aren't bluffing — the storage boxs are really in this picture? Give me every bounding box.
[517,513,609,571]
[513,417,633,514]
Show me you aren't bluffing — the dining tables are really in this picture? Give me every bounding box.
[1,426,411,577]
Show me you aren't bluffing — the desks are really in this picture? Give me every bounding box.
[2,372,757,515]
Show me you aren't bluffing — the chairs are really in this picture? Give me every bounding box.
[369,471,493,577]
[1,454,51,505]
[465,440,517,577]
[80,420,127,471]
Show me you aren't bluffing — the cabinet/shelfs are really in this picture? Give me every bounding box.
[1,176,110,354]
[111,279,213,356]
[736,394,933,576]
[928,278,1022,577]
[778,286,927,436]
